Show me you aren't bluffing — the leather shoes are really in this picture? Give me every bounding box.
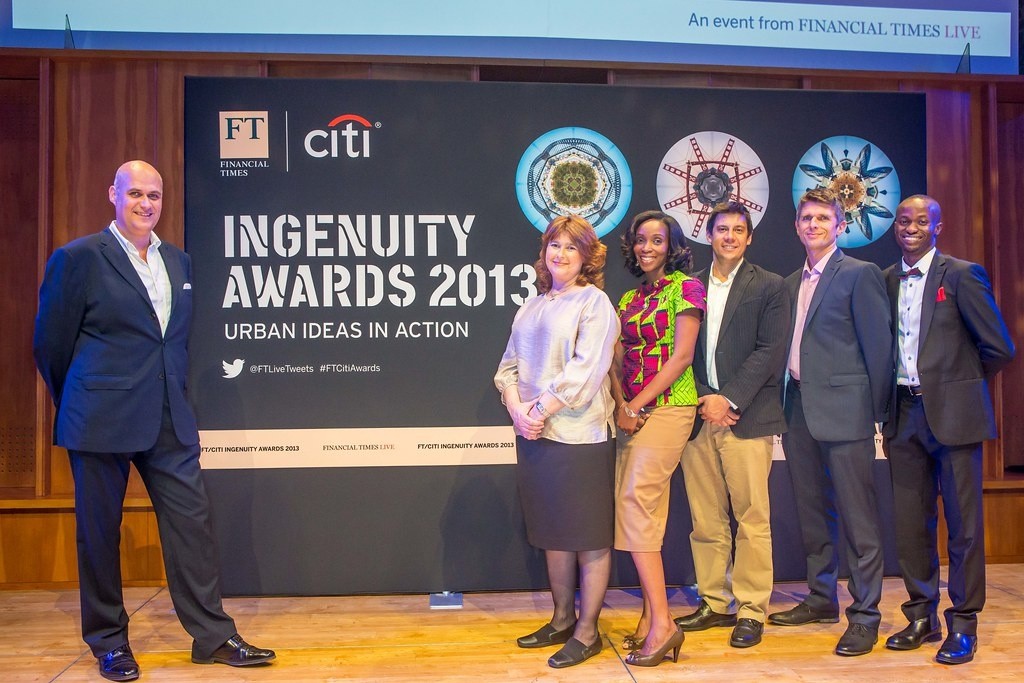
[836,623,878,654]
[936,632,978,664]
[673,600,737,631]
[730,617,764,646]
[768,602,839,626]
[548,631,602,667]
[192,633,276,665]
[886,615,942,650]
[99,644,139,680]
[517,620,577,648]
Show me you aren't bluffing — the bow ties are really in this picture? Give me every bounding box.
[898,267,922,280]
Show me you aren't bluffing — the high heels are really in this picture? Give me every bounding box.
[622,622,685,667]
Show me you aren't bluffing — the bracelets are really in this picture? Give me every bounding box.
[619,401,627,407]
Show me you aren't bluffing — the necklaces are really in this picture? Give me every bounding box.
[549,275,583,301]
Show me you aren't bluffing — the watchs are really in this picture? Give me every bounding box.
[624,406,638,418]
[535,401,552,418]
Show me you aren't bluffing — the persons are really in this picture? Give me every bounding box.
[672,203,792,648]
[32,161,276,681]
[494,216,622,667]
[768,188,895,656]
[880,195,1015,665]
[609,211,706,666]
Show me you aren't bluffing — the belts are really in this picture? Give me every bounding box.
[789,374,801,390]
[896,384,922,397]
